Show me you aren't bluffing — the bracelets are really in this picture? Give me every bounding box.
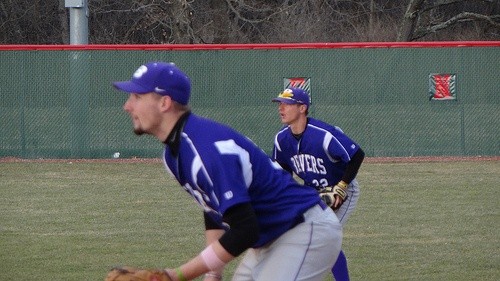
[338,181,348,190]
[204,273,222,278]
[201,244,225,271]
[175,267,187,280]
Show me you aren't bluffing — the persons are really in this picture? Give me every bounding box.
[102,61,342,280]
[272,86,364,280]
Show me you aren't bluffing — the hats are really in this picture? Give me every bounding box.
[272,87,309,105]
[112,62,190,105]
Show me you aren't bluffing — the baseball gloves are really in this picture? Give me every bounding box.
[319,186,349,214]
[104,267,179,281]
